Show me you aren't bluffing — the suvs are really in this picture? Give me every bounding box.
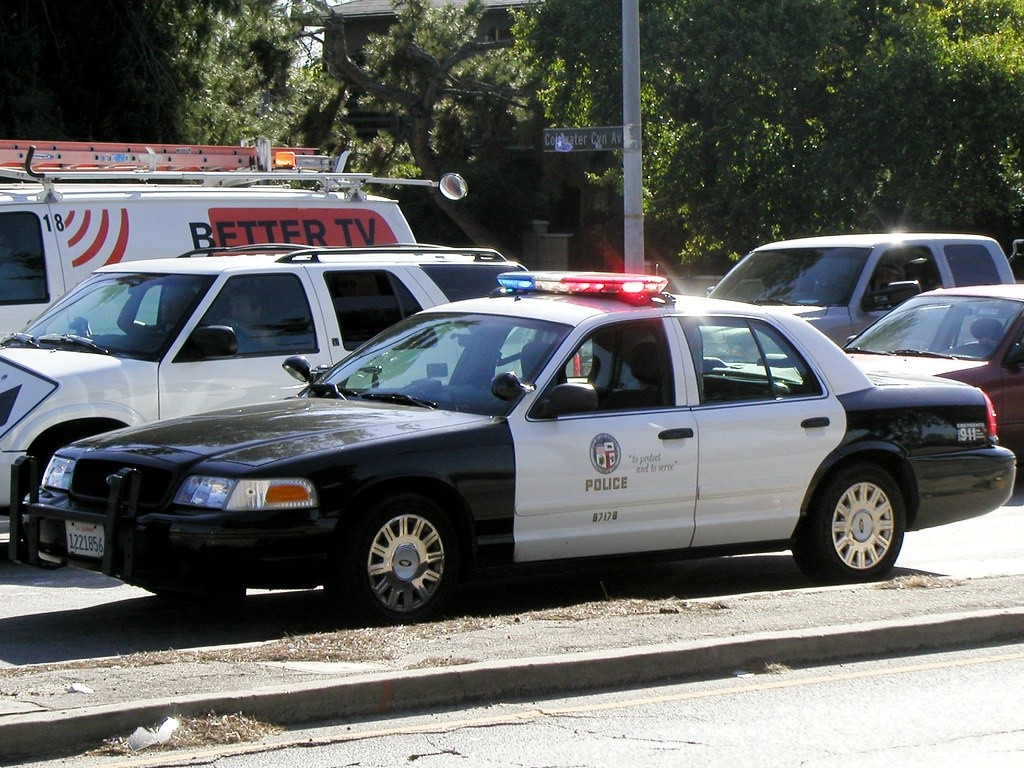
[0,241,581,550]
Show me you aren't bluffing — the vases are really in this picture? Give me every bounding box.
[532,220,549,233]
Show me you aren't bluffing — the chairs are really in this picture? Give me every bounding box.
[238,299,308,352]
[188,289,231,324]
[596,341,673,411]
[432,267,469,296]
[521,342,568,390]
[970,318,1002,341]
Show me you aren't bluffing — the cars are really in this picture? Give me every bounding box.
[840,283,1024,461]
[38,269,1016,643]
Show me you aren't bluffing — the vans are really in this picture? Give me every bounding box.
[704,232,1017,363]
[0,139,418,338]
